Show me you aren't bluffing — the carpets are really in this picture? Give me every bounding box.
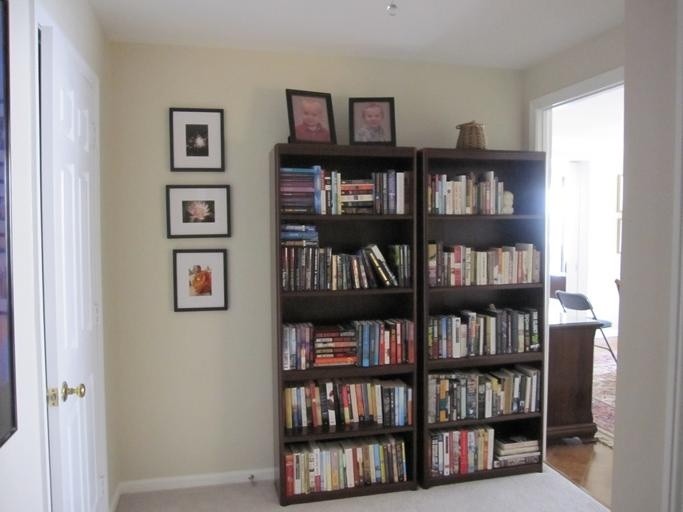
[118,464,608,510]
[592,337,618,448]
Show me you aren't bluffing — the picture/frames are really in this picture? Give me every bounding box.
[285,87,395,143]
[165,108,233,312]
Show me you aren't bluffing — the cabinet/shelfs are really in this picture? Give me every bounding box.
[419,148,545,481]
[272,144,421,501]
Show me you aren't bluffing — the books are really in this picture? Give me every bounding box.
[428,366,541,422]
[428,169,516,215]
[281,317,414,434]
[427,239,541,286]
[282,435,407,495]
[427,304,540,359]
[278,164,411,291]
[427,426,541,477]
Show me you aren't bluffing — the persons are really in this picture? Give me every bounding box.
[291,96,330,144]
[353,103,391,143]
